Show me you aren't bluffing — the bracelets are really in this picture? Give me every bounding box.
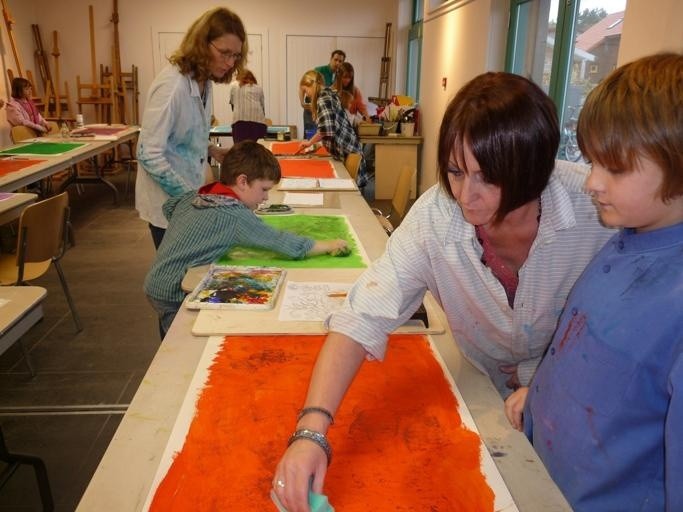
[296,407,335,425]
[286,429,332,467]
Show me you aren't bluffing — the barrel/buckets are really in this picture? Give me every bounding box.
[384,121,398,135]
[401,122,415,136]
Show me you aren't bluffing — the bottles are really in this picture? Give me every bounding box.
[61,123,69,139]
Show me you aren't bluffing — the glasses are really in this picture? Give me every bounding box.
[209,41,243,61]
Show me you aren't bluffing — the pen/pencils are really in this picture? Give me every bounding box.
[327,293,347,297]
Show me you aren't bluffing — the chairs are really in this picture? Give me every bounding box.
[0,191,83,379]
[370,166,415,236]
[346,153,362,182]
[11,126,38,145]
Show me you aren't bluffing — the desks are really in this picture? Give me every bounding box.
[181,159,389,293]
[26,123,142,201]
[74,293,576,512]
[0,284,55,512]
[359,135,423,200]
[0,193,38,254]
[0,141,119,205]
[210,125,295,168]
[256,135,333,156]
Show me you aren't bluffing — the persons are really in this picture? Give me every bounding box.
[142,140,349,333]
[330,62,372,135]
[272,71,620,511]
[229,69,266,145]
[315,50,346,87]
[503,52,683,512]
[298,70,364,182]
[134,6,249,342]
[6,77,53,194]
